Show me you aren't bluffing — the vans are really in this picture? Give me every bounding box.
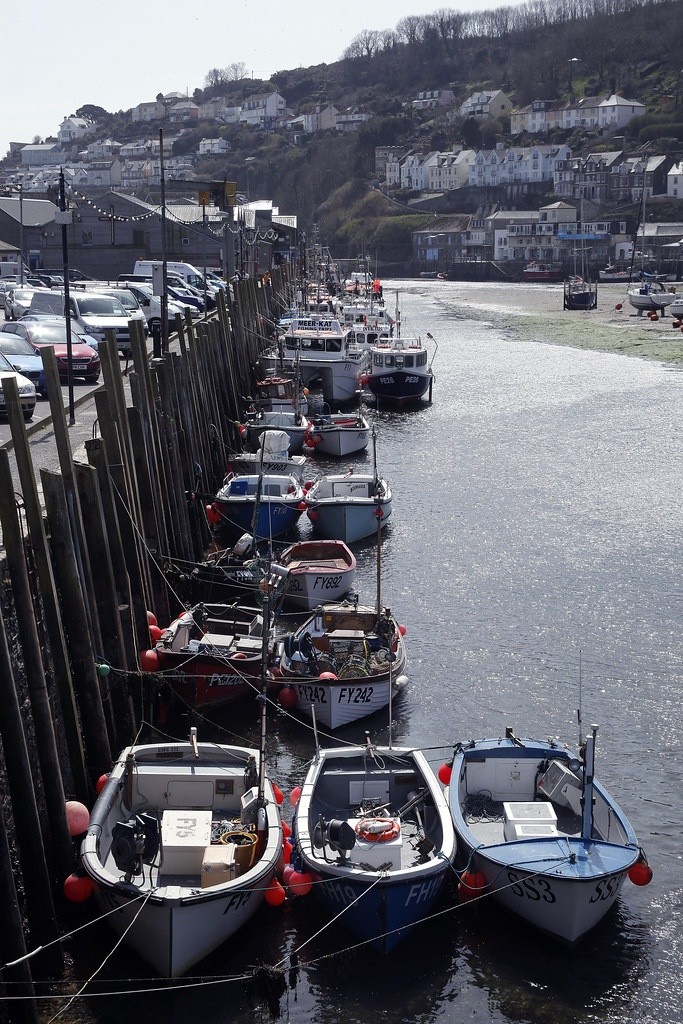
[118,274,202,299]
[26,290,134,359]
[133,259,224,302]
[74,282,186,335]
[53,287,149,342]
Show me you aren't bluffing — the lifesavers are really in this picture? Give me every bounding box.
[355,817,401,842]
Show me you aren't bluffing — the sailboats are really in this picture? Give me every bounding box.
[563,185,601,314]
[601,155,683,322]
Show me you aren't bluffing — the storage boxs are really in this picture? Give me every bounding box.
[201,843,239,887]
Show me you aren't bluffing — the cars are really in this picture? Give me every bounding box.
[148,283,200,318]
[17,313,100,359]
[0,258,96,319]
[166,285,205,312]
[0,351,36,421]
[1,320,103,383]
[0,331,50,397]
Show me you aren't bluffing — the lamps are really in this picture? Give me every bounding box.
[567,758,581,772]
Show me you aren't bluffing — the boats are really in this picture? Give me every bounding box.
[118,237,437,729]
[291,657,459,954]
[49,596,287,989]
[448,652,651,946]
[522,261,563,282]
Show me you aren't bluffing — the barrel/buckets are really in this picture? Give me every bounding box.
[220,831,258,873]
[230,481,249,494]
[226,835,253,845]
